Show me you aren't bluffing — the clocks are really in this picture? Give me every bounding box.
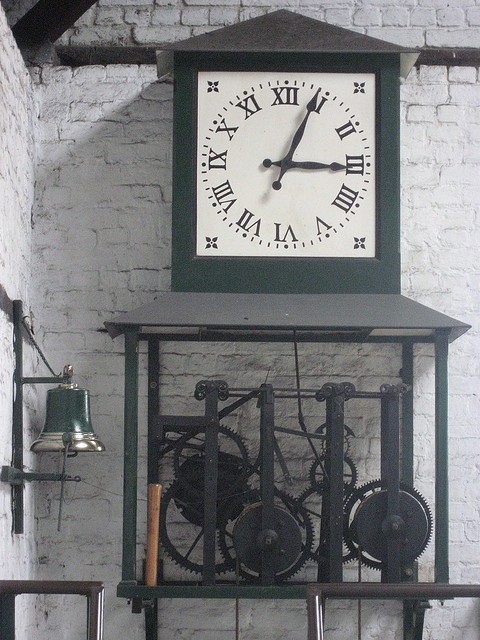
[156,9,421,292]
